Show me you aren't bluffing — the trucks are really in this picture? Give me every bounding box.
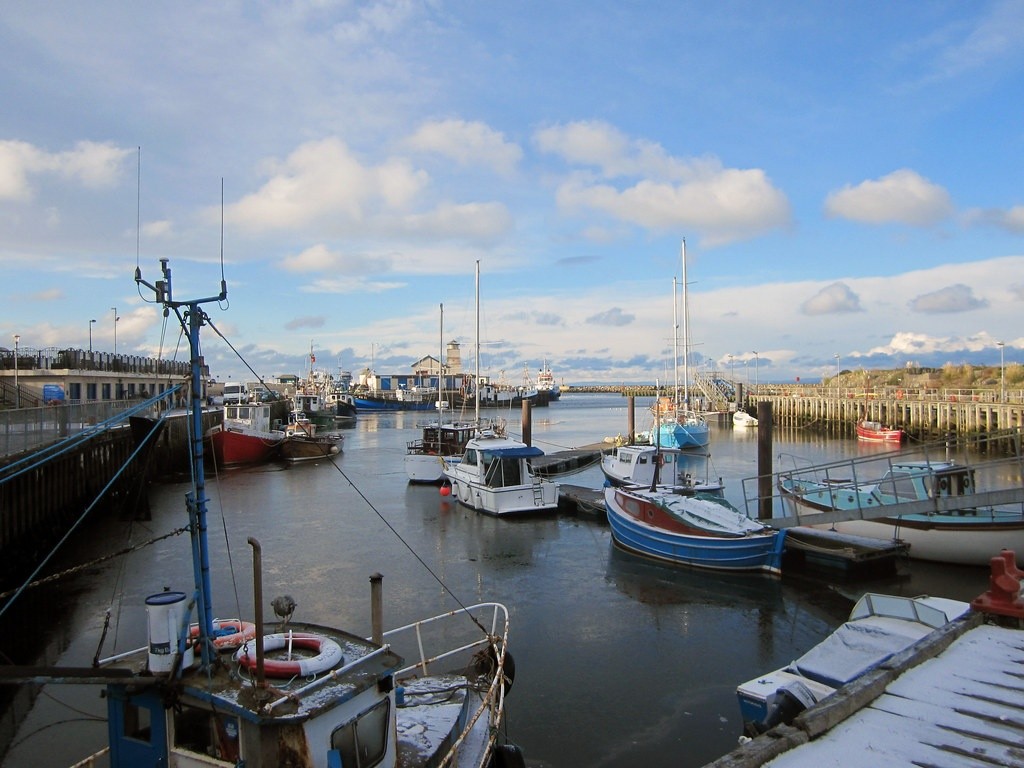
[220,382,249,405]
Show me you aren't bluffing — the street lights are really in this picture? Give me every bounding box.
[752,350,760,394]
[111,307,120,356]
[996,340,1005,404]
[11,334,21,408]
[89,320,96,352]
[727,354,734,390]
[834,352,841,399]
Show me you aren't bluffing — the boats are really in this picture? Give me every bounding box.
[271,337,357,462]
[777,452,1024,569]
[0,143,512,768]
[855,416,903,444]
[204,383,287,471]
[599,377,789,577]
[734,589,973,741]
[733,408,759,427]
[598,435,727,494]
[352,343,437,411]
[456,358,562,409]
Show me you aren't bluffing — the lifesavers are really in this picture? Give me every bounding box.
[185,620,256,653]
[235,633,343,678]
[288,430,294,437]
[896,391,903,399]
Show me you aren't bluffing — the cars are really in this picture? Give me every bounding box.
[260,390,279,403]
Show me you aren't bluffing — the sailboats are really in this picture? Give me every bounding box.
[643,236,711,450]
[441,258,561,517]
[402,302,503,483]
